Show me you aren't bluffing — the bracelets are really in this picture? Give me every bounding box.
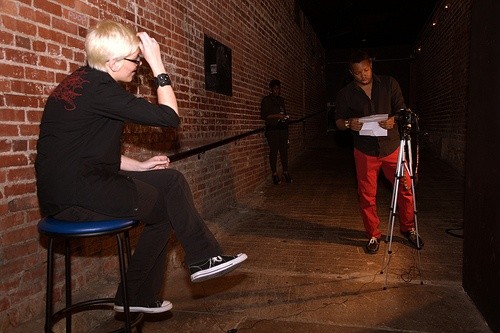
[344,120,350,129]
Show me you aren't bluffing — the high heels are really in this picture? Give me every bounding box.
[271,174,282,185]
[282,172,295,183]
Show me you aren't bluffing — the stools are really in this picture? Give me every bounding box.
[37,217,144,333]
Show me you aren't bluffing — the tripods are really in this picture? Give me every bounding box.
[379,131,425,290]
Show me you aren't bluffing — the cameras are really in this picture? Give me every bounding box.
[395,108,414,131]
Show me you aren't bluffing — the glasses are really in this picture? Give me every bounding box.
[106,55,140,67]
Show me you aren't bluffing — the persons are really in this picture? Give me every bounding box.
[34,20,249,313]
[260,80,294,184]
[333,51,424,254]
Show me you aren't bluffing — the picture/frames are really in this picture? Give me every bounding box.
[203,33,232,96]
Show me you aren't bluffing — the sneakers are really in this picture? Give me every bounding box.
[188,253,248,282]
[366,237,380,253]
[114,301,172,313]
[400,228,425,247]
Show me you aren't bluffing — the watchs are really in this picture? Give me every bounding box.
[153,74,171,89]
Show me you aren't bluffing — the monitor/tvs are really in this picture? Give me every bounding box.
[203,33,233,96]
